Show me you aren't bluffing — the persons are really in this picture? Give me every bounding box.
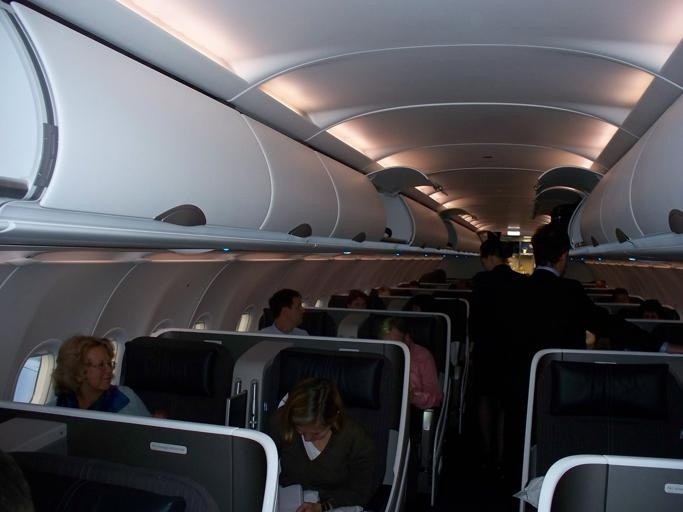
[511,221,682,353]
[468,239,528,468]
[371,316,443,410]
[409,294,436,312]
[611,287,630,303]
[639,299,666,320]
[266,376,376,512]
[344,288,370,309]
[49,335,168,419]
[255,288,310,336]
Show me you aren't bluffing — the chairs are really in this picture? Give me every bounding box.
[519,280,683,511]
[0,277,475,511]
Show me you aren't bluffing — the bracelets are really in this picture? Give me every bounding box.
[319,500,330,512]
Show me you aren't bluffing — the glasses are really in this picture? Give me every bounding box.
[89,362,116,370]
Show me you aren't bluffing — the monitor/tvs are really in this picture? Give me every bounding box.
[506,226,521,236]
